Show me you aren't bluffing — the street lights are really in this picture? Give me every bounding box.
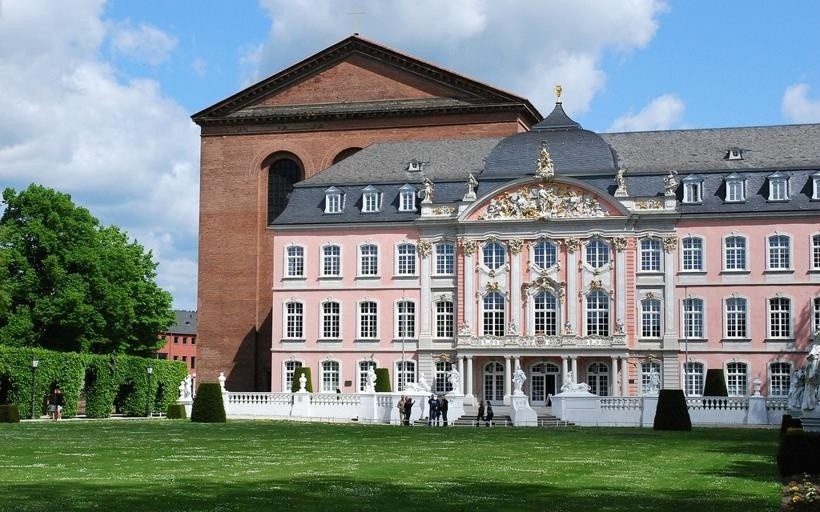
[30,356,39,420]
[145,363,154,417]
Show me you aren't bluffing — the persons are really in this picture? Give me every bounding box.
[47,389,57,419]
[55,390,63,421]
[476,401,486,427]
[485,401,495,427]
[397,395,448,427]
